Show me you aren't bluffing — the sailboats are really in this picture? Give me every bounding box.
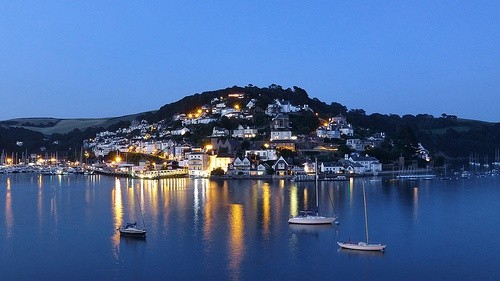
[336,180,387,252]
[288,158,339,225]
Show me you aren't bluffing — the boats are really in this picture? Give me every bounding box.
[119,222,147,236]
